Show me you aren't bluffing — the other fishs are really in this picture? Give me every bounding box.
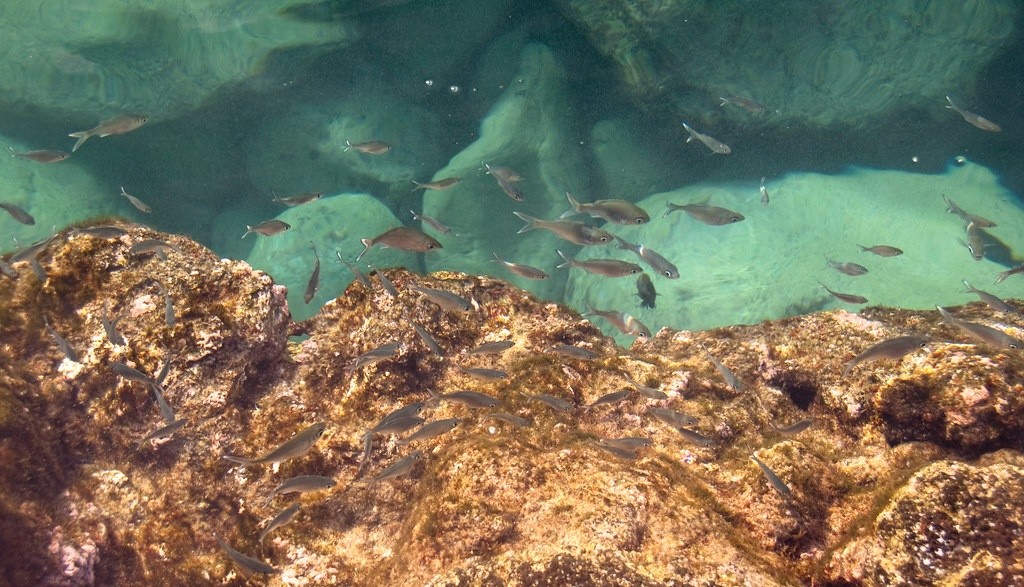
[1,90,1024,587]
